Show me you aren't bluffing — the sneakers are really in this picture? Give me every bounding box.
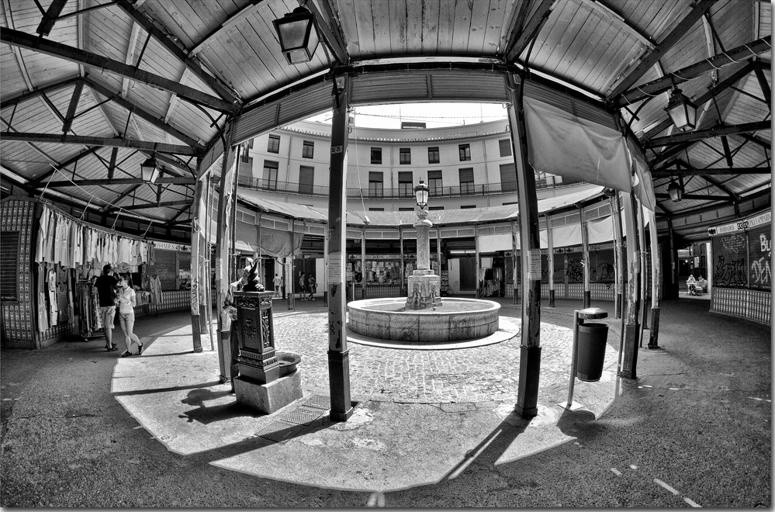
[121,350,132,358]
[104,343,118,352]
[137,343,143,355]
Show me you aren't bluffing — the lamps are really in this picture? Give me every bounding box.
[413,180,429,210]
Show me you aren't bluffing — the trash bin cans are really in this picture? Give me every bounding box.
[577,307,609,382]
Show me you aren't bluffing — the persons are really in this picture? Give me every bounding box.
[308,273,316,301]
[272,274,283,297]
[686,274,704,295]
[94,264,144,357]
[299,272,308,301]
